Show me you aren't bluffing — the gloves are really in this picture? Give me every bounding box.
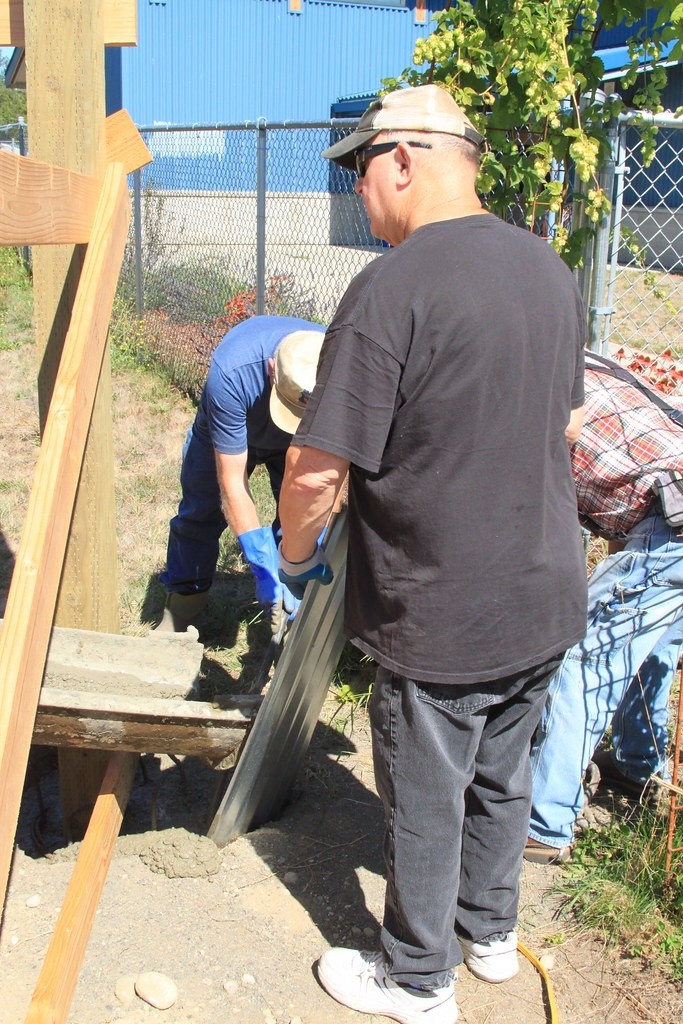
[273,525,328,620]
[237,526,296,634]
[278,536,334,599]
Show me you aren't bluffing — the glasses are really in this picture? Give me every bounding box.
[352,140,432,178]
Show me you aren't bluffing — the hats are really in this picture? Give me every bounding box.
[322,84,486,170]
[269,330,327,435]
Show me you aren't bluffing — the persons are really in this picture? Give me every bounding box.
[153,316,348,636]
[521,352,683,863]
[278,86,588,1024]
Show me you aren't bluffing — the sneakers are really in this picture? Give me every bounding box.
[453,927,520,984]
[318,948,458,1023]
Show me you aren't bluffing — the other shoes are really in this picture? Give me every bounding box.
[588,750,662,803]
[522,837,572,863]
[151,585,212,637]
